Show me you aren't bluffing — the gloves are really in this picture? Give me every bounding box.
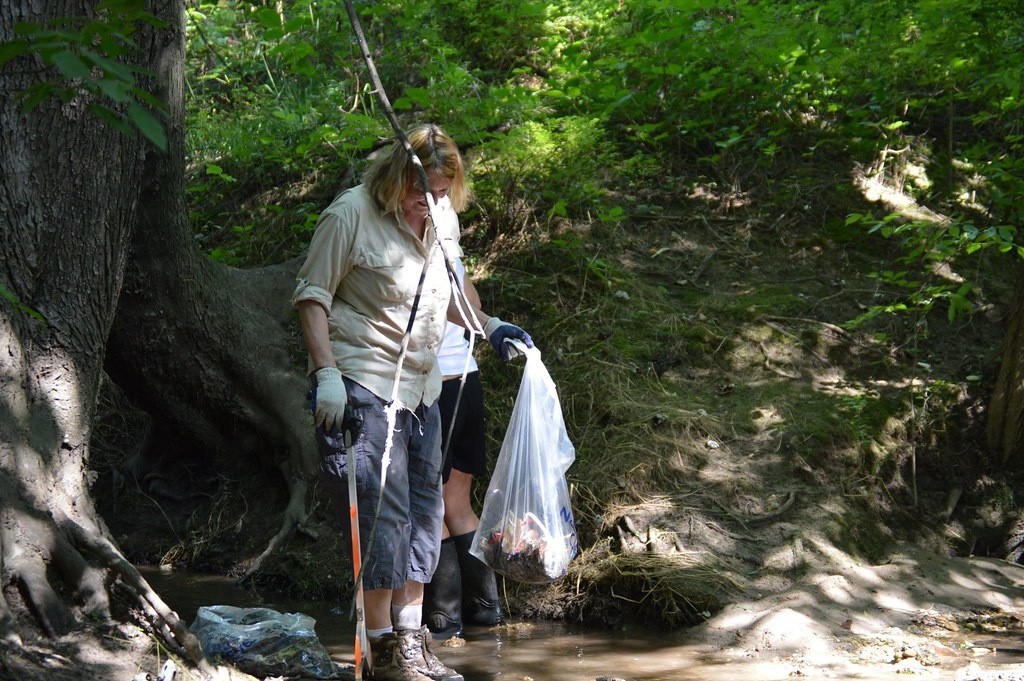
[484,318,534,363]
[315,368,348,435]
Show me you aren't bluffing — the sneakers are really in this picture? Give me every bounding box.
[397,625,463,681]
[362,633,433,681]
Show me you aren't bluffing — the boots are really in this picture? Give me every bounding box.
[423,537,463,640]
[454,531,504,626]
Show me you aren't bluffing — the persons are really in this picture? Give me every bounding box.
[426,257,503,638]
[291,122,532,681]
